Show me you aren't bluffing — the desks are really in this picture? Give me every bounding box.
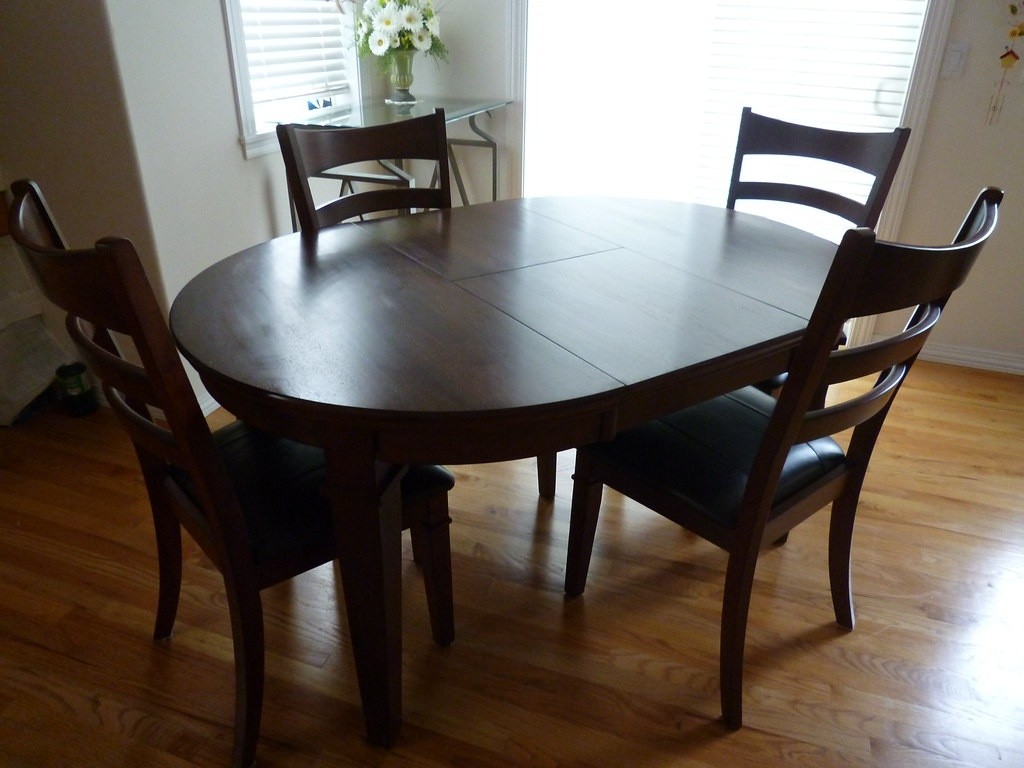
[285,96,514,234]
[169,194,849,751]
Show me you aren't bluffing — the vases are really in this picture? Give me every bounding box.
[385,48,417,105]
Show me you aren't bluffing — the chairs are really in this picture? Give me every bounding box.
[561,186,1008,730]
[726,107,913,230]
[275,106,454,231]
[6,177,460,768]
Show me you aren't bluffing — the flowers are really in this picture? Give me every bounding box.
[323,0,455,73]
